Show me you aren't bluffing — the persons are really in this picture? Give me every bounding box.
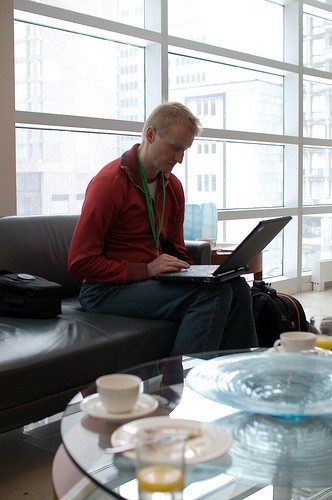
[64,102,259,384]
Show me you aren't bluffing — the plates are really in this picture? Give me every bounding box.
[199,411,332,488]
[186,351,332,421]
[111,419,232,463]
[80,392,159,422]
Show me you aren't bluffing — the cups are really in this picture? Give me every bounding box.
[136,433,185,500]
[96,373,142,413]
[274,331,317,351]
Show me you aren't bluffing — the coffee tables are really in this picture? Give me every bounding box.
[52,348,332,500]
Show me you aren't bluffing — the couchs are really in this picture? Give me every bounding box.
[0,215,211,434]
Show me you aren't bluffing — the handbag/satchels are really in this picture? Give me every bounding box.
[250,280,307,348]
[0,269,61,319]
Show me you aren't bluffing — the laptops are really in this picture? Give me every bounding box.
[150,216,291,282]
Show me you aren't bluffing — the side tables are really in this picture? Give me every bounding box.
[210,243,263,283]
